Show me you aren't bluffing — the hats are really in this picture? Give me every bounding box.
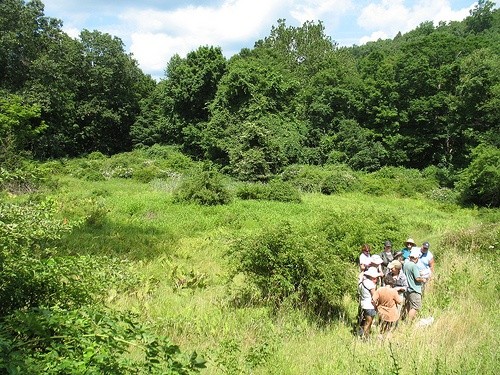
[422,242,430,249]
[370,254,384,265]
[394,251,404,259]
[363,267,382,278]
[403,239,417,247]
[385,241,391,247]
[409,251,420,258]
[388,260,402,269]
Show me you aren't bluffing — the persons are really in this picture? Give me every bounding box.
[384,260,407,319]
[401,238,434,324]
[381,241,404,287]
[353,254,384,336]
[371,276,404,335]
[357,244,370,304]
